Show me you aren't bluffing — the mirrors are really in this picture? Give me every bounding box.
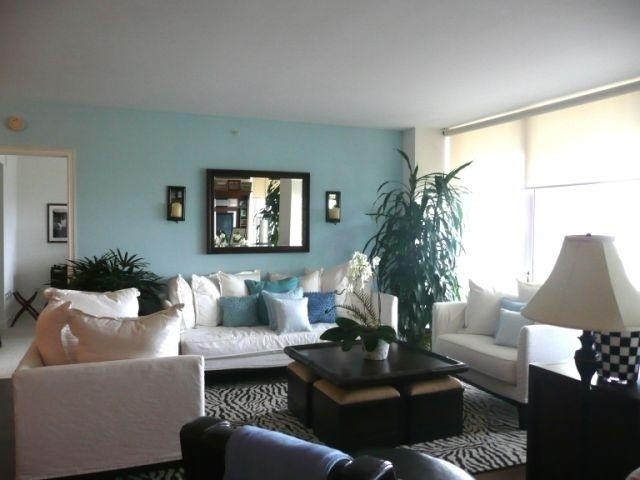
[206,168,310,253]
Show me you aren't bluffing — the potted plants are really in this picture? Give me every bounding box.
[361,143,474,353]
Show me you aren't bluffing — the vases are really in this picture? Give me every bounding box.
[362,337,390,361]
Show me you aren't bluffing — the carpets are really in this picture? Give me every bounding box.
[71,367,527,480]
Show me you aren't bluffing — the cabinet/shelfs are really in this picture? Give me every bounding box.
[524,360,640,480]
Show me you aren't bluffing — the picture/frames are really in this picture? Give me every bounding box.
[227,180,242,192]
[47,203,69,243]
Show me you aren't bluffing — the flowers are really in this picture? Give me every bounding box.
[318,251,397,353]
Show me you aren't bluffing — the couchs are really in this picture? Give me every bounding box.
[11,338,205,479]
[159,290,400,372]
[431,296,600,431]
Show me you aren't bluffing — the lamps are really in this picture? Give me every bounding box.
[325,190,341,225]
[167,185,186,224]
[520,233,640,480]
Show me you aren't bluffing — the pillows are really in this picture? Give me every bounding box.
[44,283,139,360]
[217,293,263,327]
[216,269,258,295]
[244,276,294,295]
[71,303,187,363]
[272,296,312,332]
[457,277,545,335]
[261,286,304,329]
[304,292,337,324]
[267,268,325,292]
[191,273,222,325]
[167,272,196,328]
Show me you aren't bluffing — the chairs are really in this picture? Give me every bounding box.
[178,415,398,480]
